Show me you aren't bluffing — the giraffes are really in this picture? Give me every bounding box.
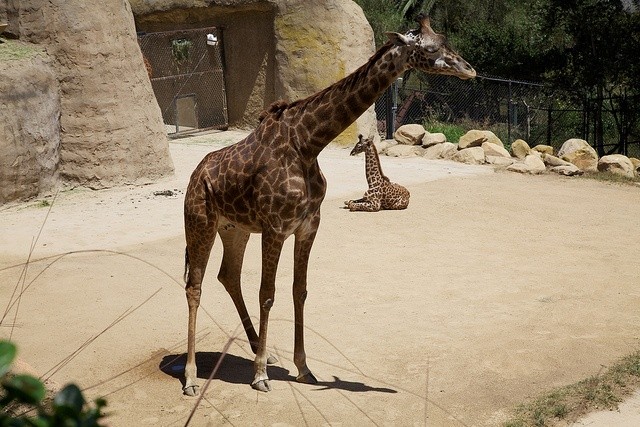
[183,12,477,397]
[343,133,410,212]
